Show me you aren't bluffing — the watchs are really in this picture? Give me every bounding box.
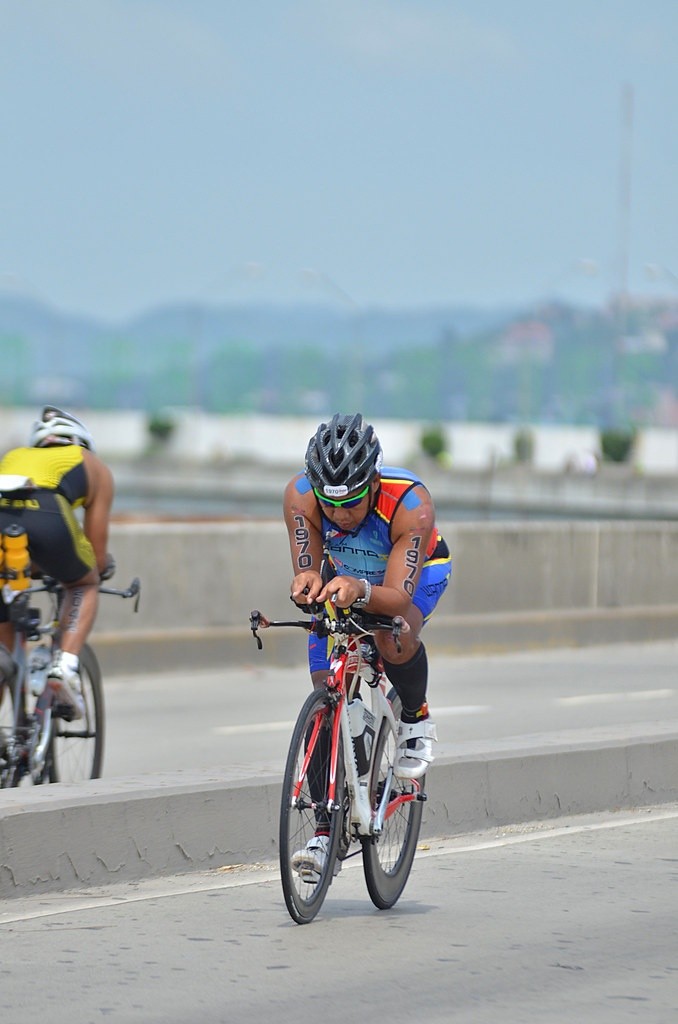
[352,578,371,609]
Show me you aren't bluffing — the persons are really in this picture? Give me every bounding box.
[285,417,451,885]
[0,407,114,719]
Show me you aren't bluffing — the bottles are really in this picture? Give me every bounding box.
[348,699,372,774]
[27,642,51,697]
[3,524,30,605]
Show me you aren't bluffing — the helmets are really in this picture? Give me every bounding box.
[304,413,383,497]
[30,405,96,454]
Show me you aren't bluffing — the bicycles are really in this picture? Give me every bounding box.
[247,595,429,925]
[0,568,141,787]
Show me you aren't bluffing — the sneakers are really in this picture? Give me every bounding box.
[393,714,438,779]
[47,664,85,720]
[290,836,342,876]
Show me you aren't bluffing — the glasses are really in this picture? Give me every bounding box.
[314,486,369,509]
